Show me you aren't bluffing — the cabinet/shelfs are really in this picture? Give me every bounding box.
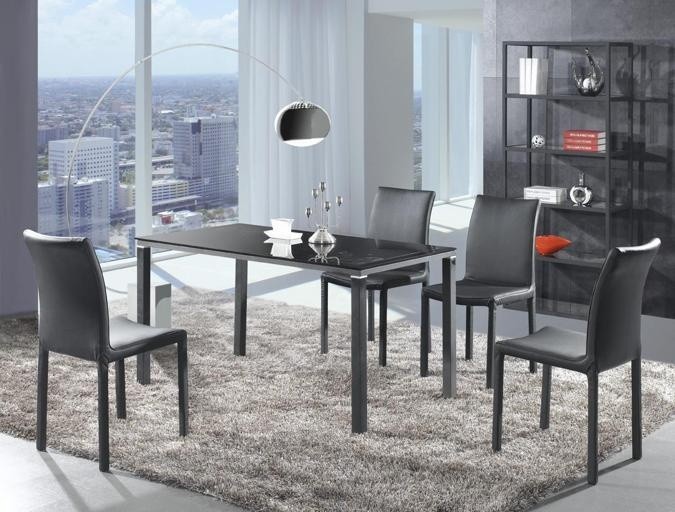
[500,41,635,322]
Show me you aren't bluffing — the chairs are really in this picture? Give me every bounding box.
[320,186,436,366]
[21,226,190,472]
[492,236,661,485]
[419,194,541,388]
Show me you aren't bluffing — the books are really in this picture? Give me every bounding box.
[563,130,606,152]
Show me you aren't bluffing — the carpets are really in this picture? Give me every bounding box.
[0,286,675,511]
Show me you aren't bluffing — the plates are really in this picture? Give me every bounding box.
[264,230,303,240]
[264,239,302,244]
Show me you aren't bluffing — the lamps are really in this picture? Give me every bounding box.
[64,43,332,240]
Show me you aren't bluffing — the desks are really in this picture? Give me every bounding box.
[132,222,459,434]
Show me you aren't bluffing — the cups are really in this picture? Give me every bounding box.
[271,218,294,234]
[270,245,295,259]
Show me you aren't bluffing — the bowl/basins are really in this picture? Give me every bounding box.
[535,235,571,257]
